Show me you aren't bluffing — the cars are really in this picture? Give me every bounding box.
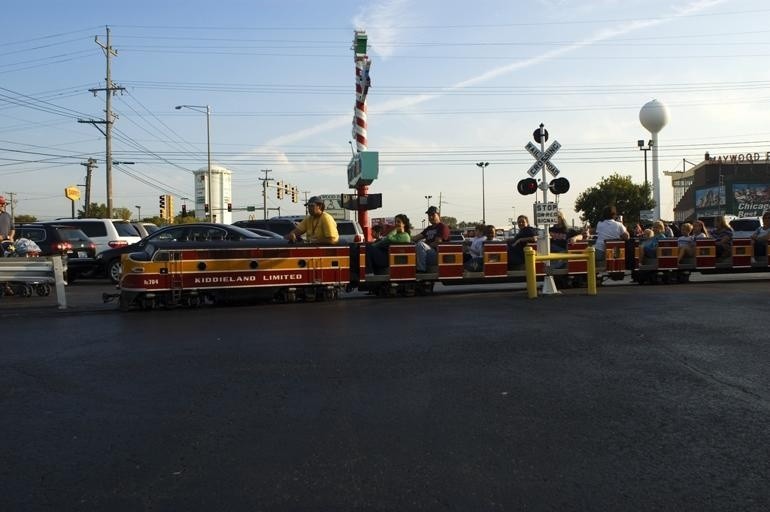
[247,227,284,241]
[93,222,266,285]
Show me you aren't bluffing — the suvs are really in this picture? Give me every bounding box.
[50,217,142,261]
[697,214,736,233]
[131,220,160,244]
[728,216,763,239]
[12,221,96,282]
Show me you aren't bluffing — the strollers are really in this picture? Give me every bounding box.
[1,237,51,298]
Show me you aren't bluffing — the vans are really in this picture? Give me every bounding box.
[232,214,395,243]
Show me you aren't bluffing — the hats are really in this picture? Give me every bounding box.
[426,206,439,213]
[304,197,323,206]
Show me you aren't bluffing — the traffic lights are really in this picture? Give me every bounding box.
[277,182,283,200]
[160,210,166,218]
[517,179,537,194]
[285,184,290,194]
[159,195,166,208]
[227,203,231,212]
[182,205,186,215]
[291,187,298,203]
[549,178,569,193]
[204,203,209,213]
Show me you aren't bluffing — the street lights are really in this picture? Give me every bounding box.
[424,195,432,228]
[638,140,655,207]
[135,205,141,220]
[476,161,489,226]
[175,103,214,222]
[640,98,667,223]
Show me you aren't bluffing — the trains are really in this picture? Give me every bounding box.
[117,238,770,310]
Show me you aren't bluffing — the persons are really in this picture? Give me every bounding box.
[594,206,630,260]
[289,196,340,246]
[365,214,412,276]
[411,205,451,274]
[626,211,770,267]
[507,211,584,276]
[458,224,504,278]
[0,196,16,295]
